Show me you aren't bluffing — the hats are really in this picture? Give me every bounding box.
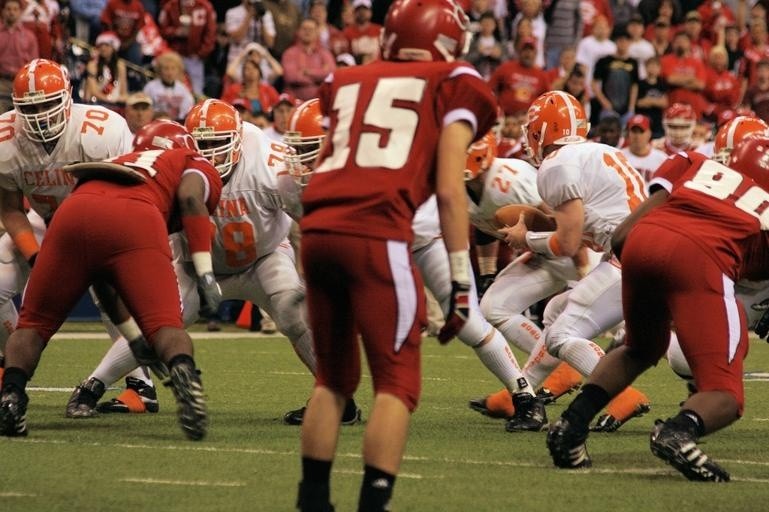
[654,16,670,25]
[627,14,642,24]
[96,30,122,51]
[229,91,295,113]
[126,92,154,106]
[686,11,702,21]
[516,36,536,48]
[352,0,371,8]
[626,114,650,131]
[717,108,755,125]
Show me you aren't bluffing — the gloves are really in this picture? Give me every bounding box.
[434,279,471,346]
[751,296,768,343]
[194,270,223,325]
[129,333,176,387]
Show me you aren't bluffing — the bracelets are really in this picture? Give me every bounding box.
[11,228,40,264]
[190,250,214,282]
[477,255,498,278]
[115,316,143,348]
[524,230,562,261]
[448,247,473,293]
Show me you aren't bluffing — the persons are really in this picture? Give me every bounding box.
[288,96,548,435]
[288,0,505,510]
[601,108,768,393]
[542,127,768,490]
[0,113,226,441]
[467,88,655,433]
[0,58,161,417]
[62,97,364,430]
[453,130,602,424]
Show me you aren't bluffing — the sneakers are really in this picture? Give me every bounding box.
[207,321,221,332]
[1,383,30,437]
[650,417,731,482]
[546,418,592,468]
[590,385,653,432]
[283,394,362,428]
[96,376,159,414]
[259,317,276,334]
[162,362,210,441]
[467,360,582,432]
[66,377,104,418]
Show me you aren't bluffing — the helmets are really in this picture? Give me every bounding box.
[383,0,471,64]
[283,97,333,187]
[183,98,243,179]
[462,127,497,182]
[663,103,695,144]
[12,59,74,143]
[131,118,198,151]
[714,116,769,194]
[520,89,589,166]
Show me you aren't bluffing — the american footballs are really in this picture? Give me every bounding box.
[494,205,551,232]
